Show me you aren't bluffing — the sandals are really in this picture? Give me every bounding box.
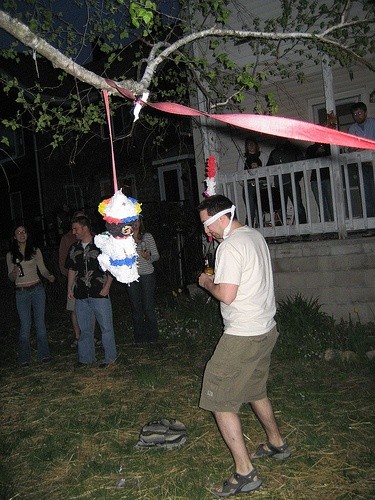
[250,441,292,459]
[215,467,261,496]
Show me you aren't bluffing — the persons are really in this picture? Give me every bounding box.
[198,195,285,493]
[129,213,159,343]
[60,212,117,368]
[6,225,55,369]
[237,102,375,228]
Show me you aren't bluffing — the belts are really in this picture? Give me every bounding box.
[16,282,40,291]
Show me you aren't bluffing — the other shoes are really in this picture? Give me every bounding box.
[99,363,107,368]
[43,359,49,363]
[74,363,86,368]
[21,363,29,368]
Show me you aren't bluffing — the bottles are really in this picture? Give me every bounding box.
[203,255,214,276]
[16,258,24,277]
